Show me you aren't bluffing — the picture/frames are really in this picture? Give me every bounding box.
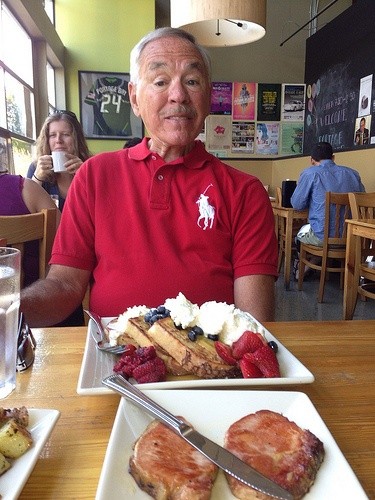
[77,70,145,141]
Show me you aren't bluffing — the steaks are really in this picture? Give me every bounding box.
[221,409,325,500]
[128,415,218,500]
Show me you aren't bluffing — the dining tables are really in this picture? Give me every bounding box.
[0,320,375,500]
[344,219,375,320]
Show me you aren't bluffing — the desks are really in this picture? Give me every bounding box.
[0,208,56,290]
[272,206,309,292]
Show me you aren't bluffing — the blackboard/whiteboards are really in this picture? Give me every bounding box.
[302,0,375,156]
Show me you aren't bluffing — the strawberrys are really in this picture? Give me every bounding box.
[214,330,280,378]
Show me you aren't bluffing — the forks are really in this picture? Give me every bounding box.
[83,309,131,355]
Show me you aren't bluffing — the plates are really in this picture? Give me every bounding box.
[76,315,315,396]
[0,407,61,500]
[95,389,369,500]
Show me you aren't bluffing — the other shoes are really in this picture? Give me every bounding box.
[293,259,300,269]
[292,268,300,281]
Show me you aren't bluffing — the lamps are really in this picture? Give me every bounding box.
[170,0,267,48]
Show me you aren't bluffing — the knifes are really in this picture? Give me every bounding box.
[102,374,293,500]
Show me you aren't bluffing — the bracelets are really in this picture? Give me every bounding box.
[33,174,43,182]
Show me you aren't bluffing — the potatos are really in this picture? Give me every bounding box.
[0,407,34,475]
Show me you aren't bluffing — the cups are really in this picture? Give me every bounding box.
[50,194,59,209]
[0,246,22,400]
[46,151,69,173]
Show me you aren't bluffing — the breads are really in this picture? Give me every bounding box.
[106,315,245,378]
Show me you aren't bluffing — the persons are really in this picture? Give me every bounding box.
[290,141,368,280]
[19,28,278,328]
[355,118,369,143]
[27,109,94,215]
[0,173,60,291]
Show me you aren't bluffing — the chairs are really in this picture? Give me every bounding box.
[348,191,375,303]
[297,192,354,303]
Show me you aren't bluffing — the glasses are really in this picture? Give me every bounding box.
[54,110,78,121]
[14,311,36,372]
